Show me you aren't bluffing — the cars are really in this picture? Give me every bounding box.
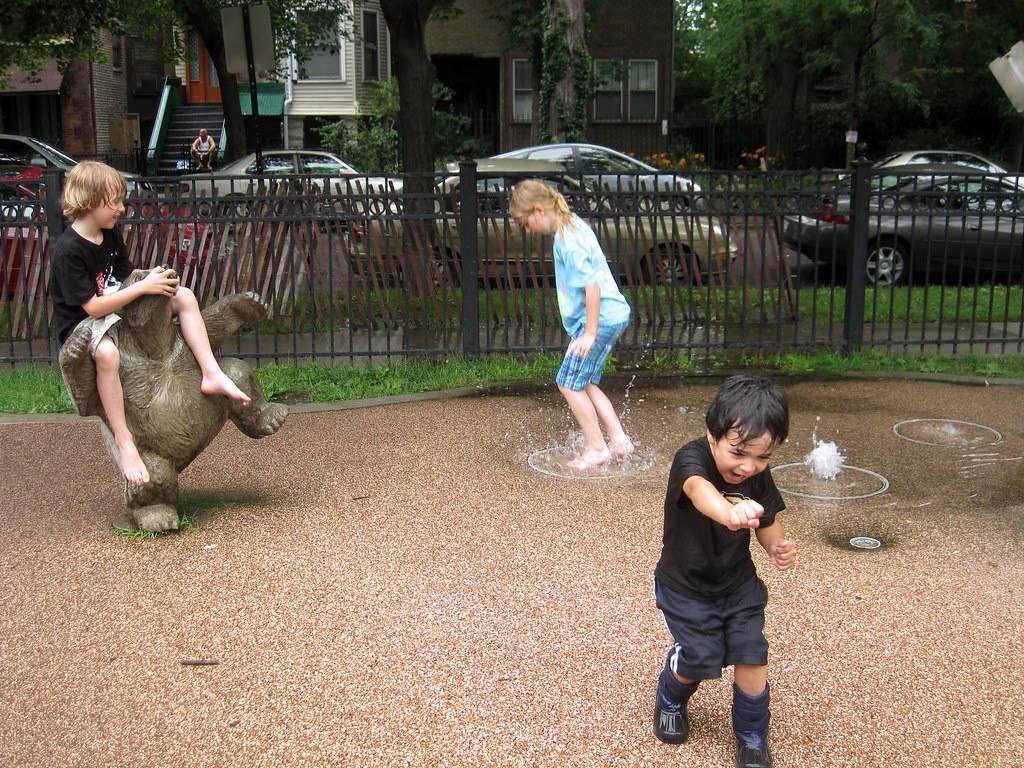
[177,149,404,218]
[346,159,741,288]
[777,163,1024,285]
[433,143,707,209]
[0,134,152,199]
[0,166,227,301]
[834,151,1023,209]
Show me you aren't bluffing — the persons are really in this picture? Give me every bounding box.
[653,374,797,768]
[191,128,216,170]
[509,181,635,470]
[50,162,251,486]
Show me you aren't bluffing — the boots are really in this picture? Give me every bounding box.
[654,647,701,744]
[732,680,771,768]
